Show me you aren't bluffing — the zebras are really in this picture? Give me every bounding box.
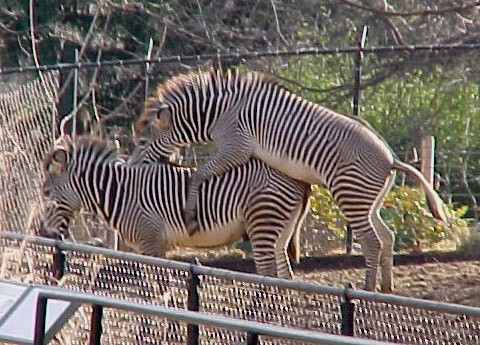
[127,62,451,296]
[39,133,314,342]
